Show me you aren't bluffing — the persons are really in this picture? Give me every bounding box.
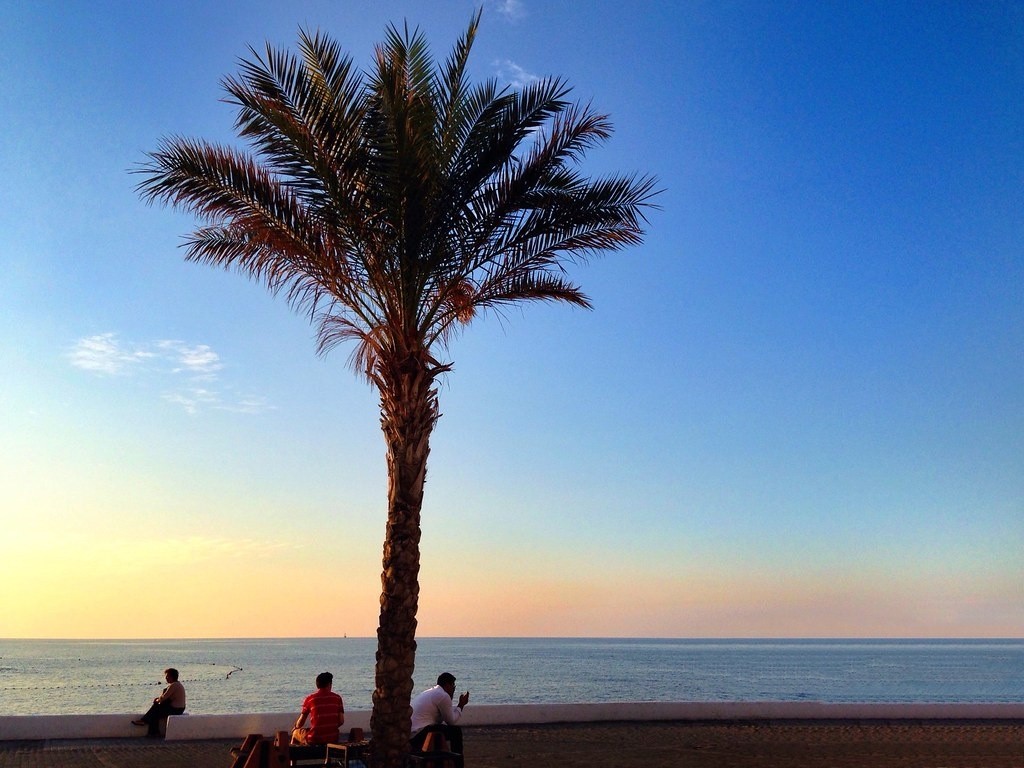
[409,672,469,768]
[131,668,185,739]
[290,672,344,766]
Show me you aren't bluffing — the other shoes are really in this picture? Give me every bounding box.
[144,733,163,739]
[132,719,147,726]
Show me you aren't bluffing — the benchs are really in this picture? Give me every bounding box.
[409,732,463,768]
[228,726,372,768]
